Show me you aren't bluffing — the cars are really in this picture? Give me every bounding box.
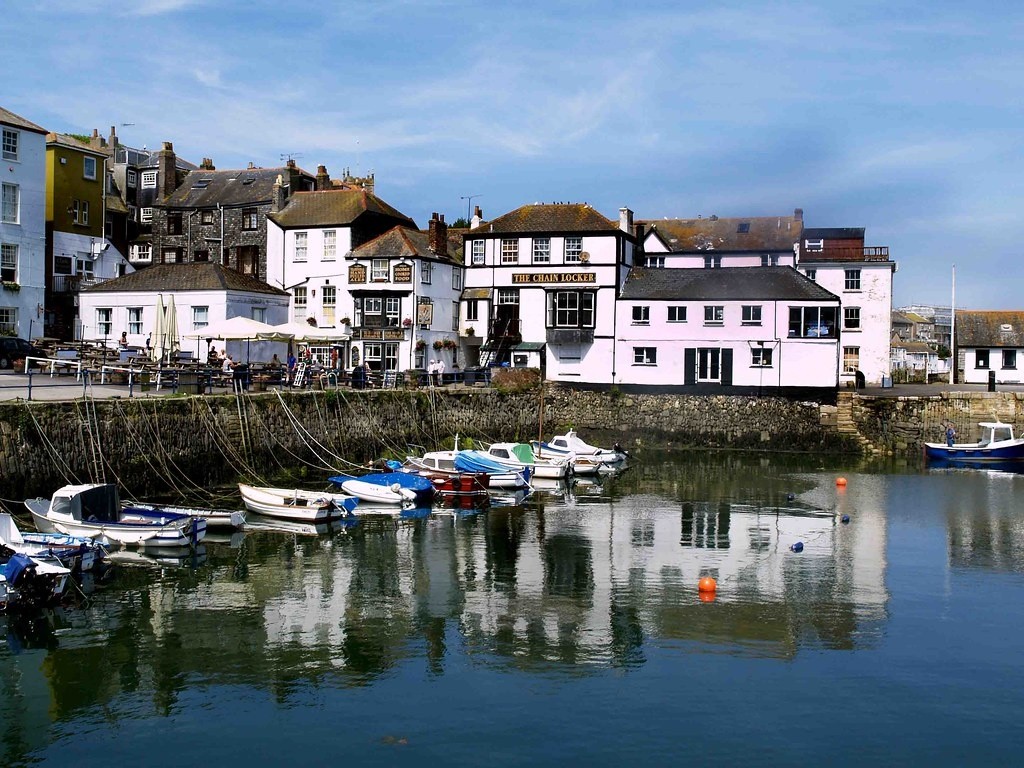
[0,336,48,370]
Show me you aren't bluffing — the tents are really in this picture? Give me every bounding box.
[259,320,353,369]
[186,316,276,370]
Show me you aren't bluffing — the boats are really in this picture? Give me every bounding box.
[474,443,577,479]
[23,483,207,547]
[120,500,248,529]
[924,422,1024,474]
[382,458,491,495]
[238,482,360,522]
[327,472,438,509]
[0,545,72,610]
[0,510,111,575]
[529,428,630,476]
[407,433,535,488]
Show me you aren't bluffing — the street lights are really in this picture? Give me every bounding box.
[949,263,956,385]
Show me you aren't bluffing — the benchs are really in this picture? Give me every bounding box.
[31,334,382,391]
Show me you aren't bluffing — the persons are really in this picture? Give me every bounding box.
[947,424,955,447]
[206,346,325,387]
[426,359,445,387]
[120,332,129,349]
[144,332,152,361]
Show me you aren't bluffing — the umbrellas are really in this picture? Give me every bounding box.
[149,294,181,368]
